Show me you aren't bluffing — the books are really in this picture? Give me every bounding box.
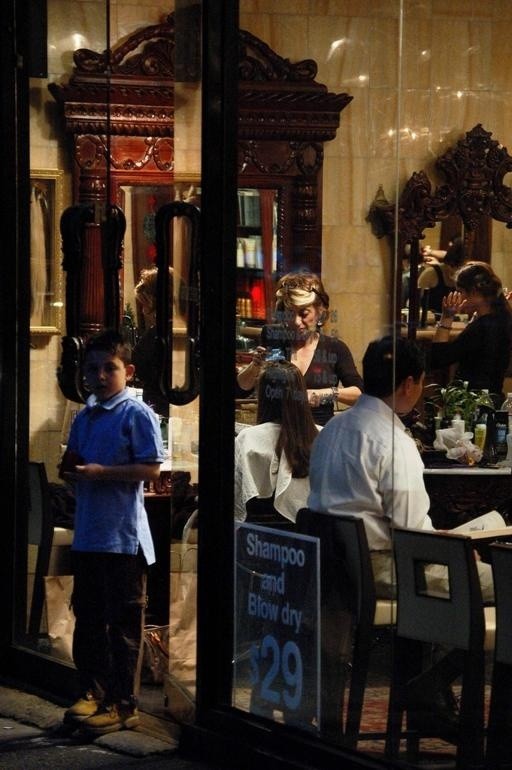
[450,509,512,540]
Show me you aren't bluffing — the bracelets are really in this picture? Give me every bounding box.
[331,386,339,401]
[435,322,454,331]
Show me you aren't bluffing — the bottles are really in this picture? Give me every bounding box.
[401,308,410,323]
[500,392,512,433]
[237,240,245,267]
[475,388,494,424]
[495,410,510,444]
[434,412,443,431]
[474,424,487,451]
[236,298,252,320]
[452,420,465,435]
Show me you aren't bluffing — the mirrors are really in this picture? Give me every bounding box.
[366,122,512,347]
[46,8,355,411]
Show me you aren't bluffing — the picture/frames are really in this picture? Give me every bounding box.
[30,168,67,337]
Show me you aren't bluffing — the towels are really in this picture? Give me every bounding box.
[433,427,483,465]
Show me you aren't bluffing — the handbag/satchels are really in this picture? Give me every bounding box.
[140,625,169,684]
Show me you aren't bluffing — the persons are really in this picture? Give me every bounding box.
[308,333,497,745]
[237,272,363,426]
[65,332,162,733]
[130,268,188,422]
[429,262,511,400]
[235,361,324,516]
[403,234,464,312]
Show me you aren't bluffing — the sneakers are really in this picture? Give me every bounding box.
[63,689,102,728]
[81,703,139,734]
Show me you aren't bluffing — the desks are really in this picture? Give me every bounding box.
[424,467,511,530]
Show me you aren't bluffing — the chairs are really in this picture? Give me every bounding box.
[243,514,298,586]
[25,462,76,652]
[487,539,512,769]
[296,508,396,749]
[380,516,497,769]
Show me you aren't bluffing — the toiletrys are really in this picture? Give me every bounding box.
[231,190,264,315]
[495,410,509,460]
[236,233,277,271]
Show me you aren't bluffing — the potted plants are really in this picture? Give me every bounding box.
[425,379,496,447]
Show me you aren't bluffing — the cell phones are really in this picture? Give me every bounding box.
[259,345,288,363]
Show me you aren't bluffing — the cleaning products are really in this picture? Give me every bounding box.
[476,404,499,464]
